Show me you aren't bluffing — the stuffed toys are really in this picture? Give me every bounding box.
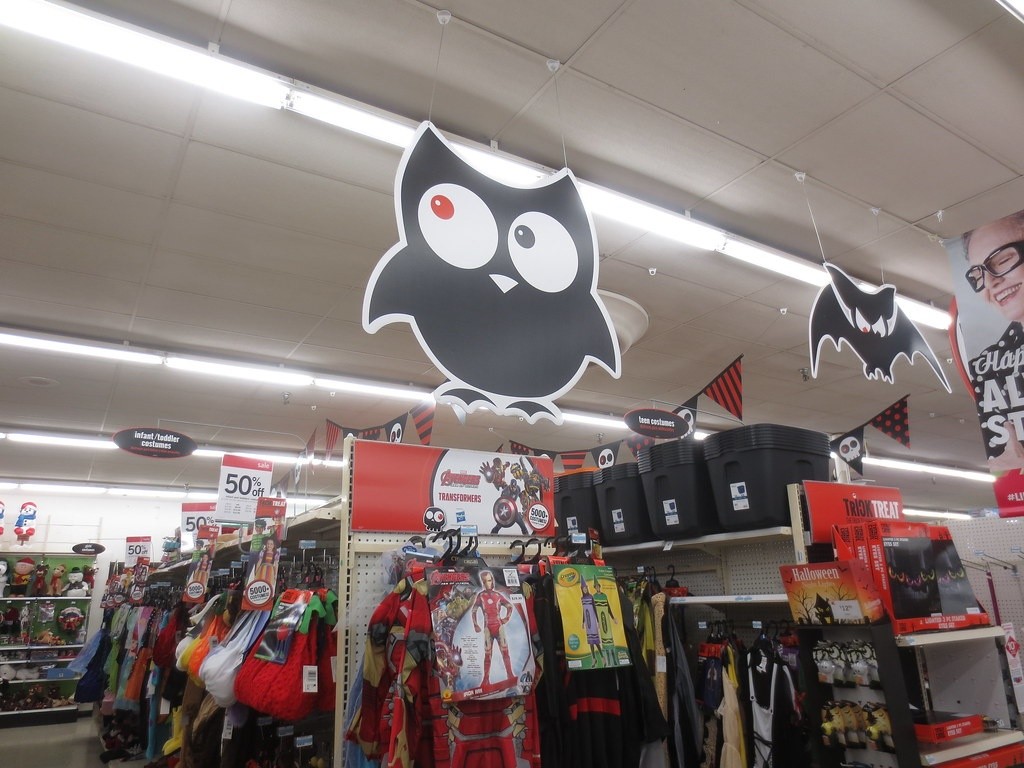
[0,501,95,685]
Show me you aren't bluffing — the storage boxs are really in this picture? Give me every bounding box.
[636,439,715,541]
[592,461,654,545]
[701,423,832,530]
[911,711,983,744]
[552,471,604,548]
[927,742,1024,768]
[839,521,981,619]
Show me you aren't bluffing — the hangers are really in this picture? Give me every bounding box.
[403,527,491,581]
[706,618,737,645]
[753,619,797,651]
[611,566,695,602]
[506,536,599,571]
[295,560,324,591]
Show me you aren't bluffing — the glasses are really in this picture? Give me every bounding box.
[965,240,1024,293]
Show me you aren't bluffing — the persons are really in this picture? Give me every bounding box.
[185,519,280,594]
[490,479,530,535]
[962,209,1024,476]
[479,457,510,492]
[471,571,516,687]
[579,574,620,668]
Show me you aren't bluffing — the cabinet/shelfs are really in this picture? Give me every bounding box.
[579,481,867,604]
[0,551,96,728]
[896,625,1024,768]
